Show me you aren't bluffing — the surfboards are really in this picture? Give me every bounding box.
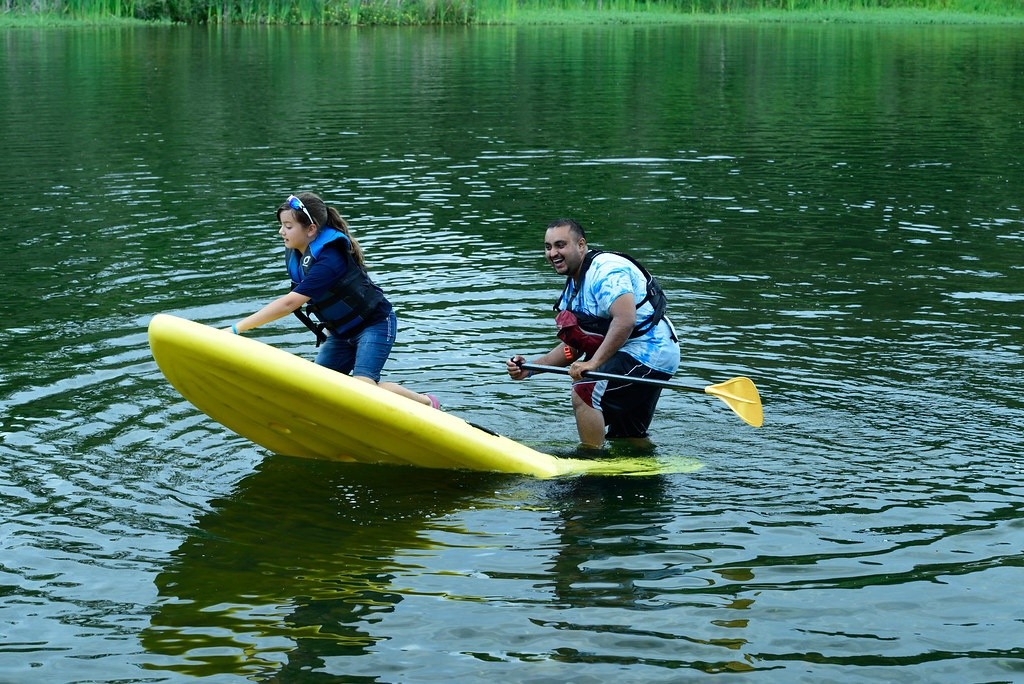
[149,311,702,482]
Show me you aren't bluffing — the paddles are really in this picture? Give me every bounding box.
[512,353,765,429]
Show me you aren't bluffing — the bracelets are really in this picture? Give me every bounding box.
[232,323,237,334]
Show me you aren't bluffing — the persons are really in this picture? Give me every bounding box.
[506,217,680,446]
[223,193,438,407]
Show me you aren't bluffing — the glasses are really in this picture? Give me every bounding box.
[286,194,314,224]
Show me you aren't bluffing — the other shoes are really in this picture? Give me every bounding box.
[423,393,440,410]
